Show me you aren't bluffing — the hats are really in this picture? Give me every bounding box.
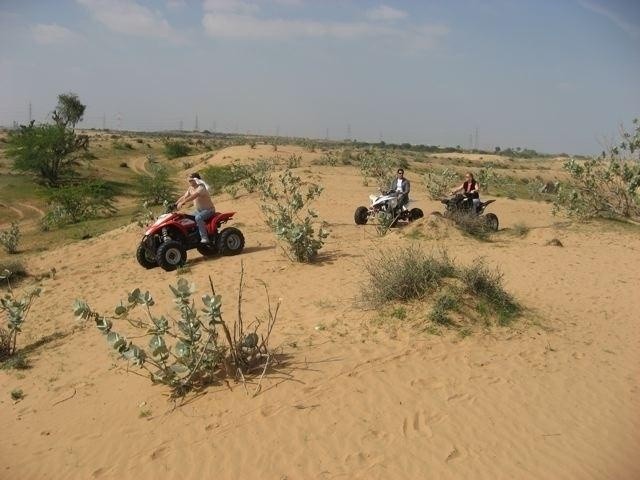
[190,173,201,179]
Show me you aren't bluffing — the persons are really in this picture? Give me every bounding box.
[175,173,215,243]
[450,172,480,214]
[390,169,410,220]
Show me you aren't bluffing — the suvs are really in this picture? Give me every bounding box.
[355,188,498,231]
[137,200,245,271]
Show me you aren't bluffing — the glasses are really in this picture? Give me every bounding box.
[399,173,403,174]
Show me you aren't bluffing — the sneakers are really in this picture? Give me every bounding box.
[200,237,209,243]
